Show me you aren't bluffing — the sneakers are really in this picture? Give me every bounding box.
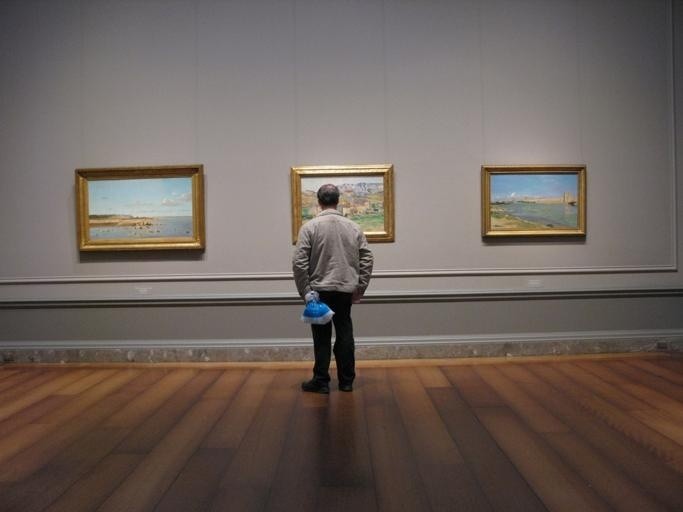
[338,383,353,392]
[301,380,329,394]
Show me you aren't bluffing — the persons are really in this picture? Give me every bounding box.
[292,185,373,395]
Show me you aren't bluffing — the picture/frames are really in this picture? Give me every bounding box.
[70,162,207,254]
[481,164,586,241]
[284,164,397,245]
